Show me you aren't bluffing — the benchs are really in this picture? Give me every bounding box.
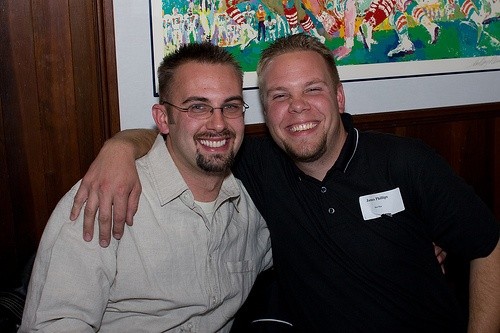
[243,101,500,228]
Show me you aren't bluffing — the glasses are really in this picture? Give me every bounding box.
[161,101,250,119]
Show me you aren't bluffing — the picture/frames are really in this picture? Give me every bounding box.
[150,1,500,97]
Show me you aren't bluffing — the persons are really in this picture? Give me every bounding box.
[18,41,447,333]
[70,33,500,333]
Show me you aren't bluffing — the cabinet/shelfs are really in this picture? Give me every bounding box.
[0,0,120,289]
